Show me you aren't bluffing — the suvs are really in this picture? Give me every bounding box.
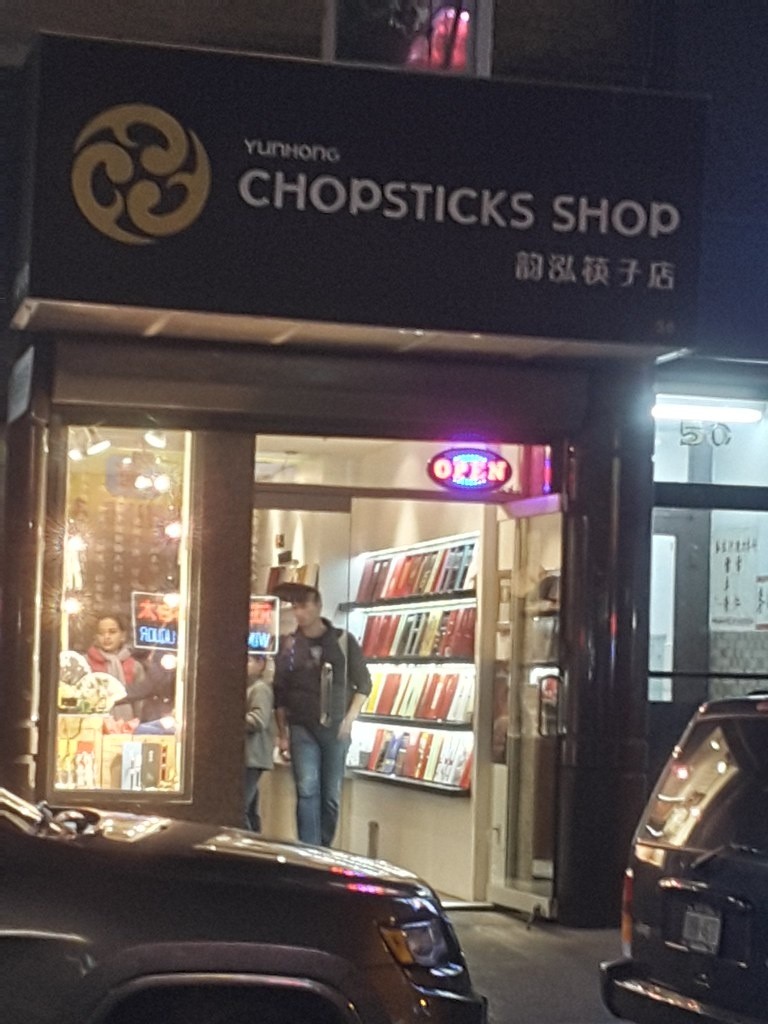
[597,687,767,1024]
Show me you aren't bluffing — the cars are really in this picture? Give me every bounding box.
[1,784,493,1024]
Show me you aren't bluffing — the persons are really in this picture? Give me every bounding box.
[272,582,372,848]
[242,643,279,833]
[81,613,148,722]
[139,618,178,723]
